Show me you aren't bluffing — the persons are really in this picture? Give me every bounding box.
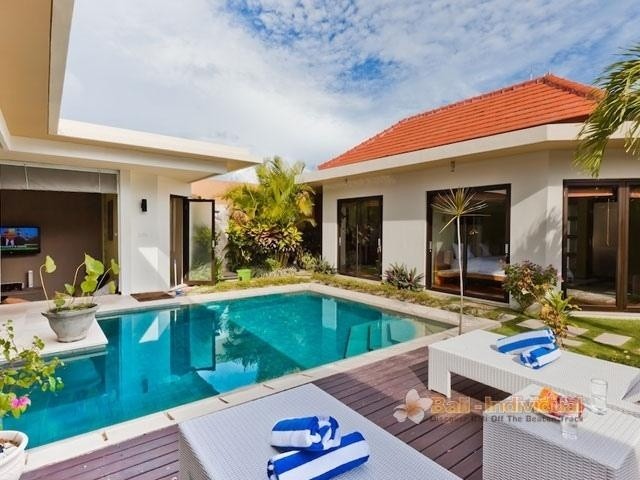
[0,228,26,246]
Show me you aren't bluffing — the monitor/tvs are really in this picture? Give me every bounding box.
[0,226,41,254]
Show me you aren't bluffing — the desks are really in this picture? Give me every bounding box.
[482,383,640,480]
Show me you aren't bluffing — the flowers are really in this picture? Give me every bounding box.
[498,255,564,311]
[0,317,68,429]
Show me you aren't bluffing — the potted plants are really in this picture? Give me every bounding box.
[37,251,121,343]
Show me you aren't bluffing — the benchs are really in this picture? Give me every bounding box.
[427,329,640,405]
[176,380,468,480]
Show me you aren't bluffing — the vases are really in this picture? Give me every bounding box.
[0,430,29,480]
[53,359,103,394]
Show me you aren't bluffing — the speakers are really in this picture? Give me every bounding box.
[28,270,33,288]
[141,198,148,211]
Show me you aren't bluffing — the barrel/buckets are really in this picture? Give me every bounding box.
[236,269,252,282]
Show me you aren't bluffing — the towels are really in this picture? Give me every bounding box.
[496,325,562,370]
[266,413,371,480]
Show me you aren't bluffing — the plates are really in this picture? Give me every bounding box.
[527,396,561,424]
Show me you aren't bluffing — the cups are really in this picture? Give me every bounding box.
[560,416,577,441]
[589,377,609,415]
